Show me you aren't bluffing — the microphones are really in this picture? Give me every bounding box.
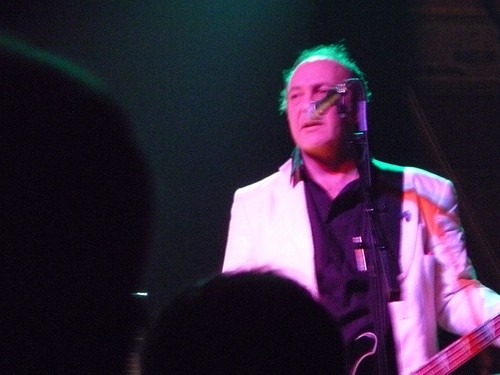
[308,79,355,119]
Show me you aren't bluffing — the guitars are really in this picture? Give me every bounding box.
[410,313,500,372]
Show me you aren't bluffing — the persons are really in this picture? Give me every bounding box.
[220,47,500,375]
[126,267,352,375]
